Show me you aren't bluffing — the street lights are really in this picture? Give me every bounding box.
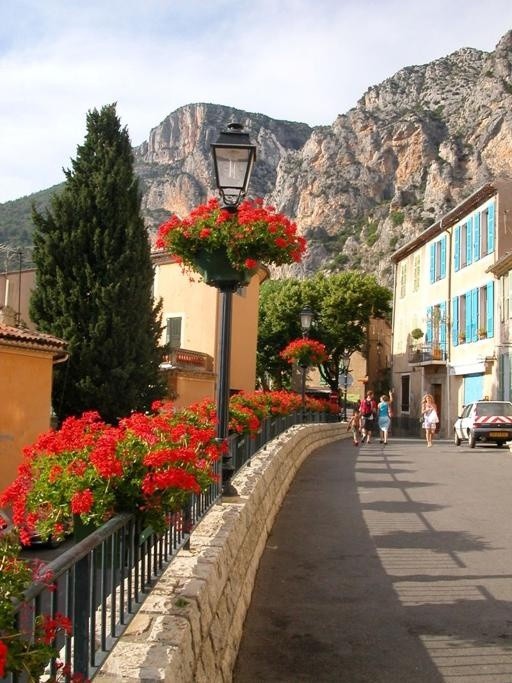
[209,121,259,500]
[298,305,316,425]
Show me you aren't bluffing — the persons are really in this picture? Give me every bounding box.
[419,393,439,448]
[345,387,395,446]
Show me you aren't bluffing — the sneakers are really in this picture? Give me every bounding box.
[361,435,366,443]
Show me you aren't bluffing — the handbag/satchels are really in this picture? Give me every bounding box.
[419,416,424,423]
[388,407,393,417]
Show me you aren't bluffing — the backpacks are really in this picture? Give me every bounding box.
[361,398,374,417]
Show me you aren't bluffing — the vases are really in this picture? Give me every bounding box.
[203,245,251,287]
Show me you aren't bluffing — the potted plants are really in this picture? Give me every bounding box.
[427,305,489,360]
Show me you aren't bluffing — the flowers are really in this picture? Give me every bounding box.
[279,338,331,367]
[148,192,309,272]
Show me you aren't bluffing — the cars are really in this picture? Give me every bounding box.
[453,399,512,448]
